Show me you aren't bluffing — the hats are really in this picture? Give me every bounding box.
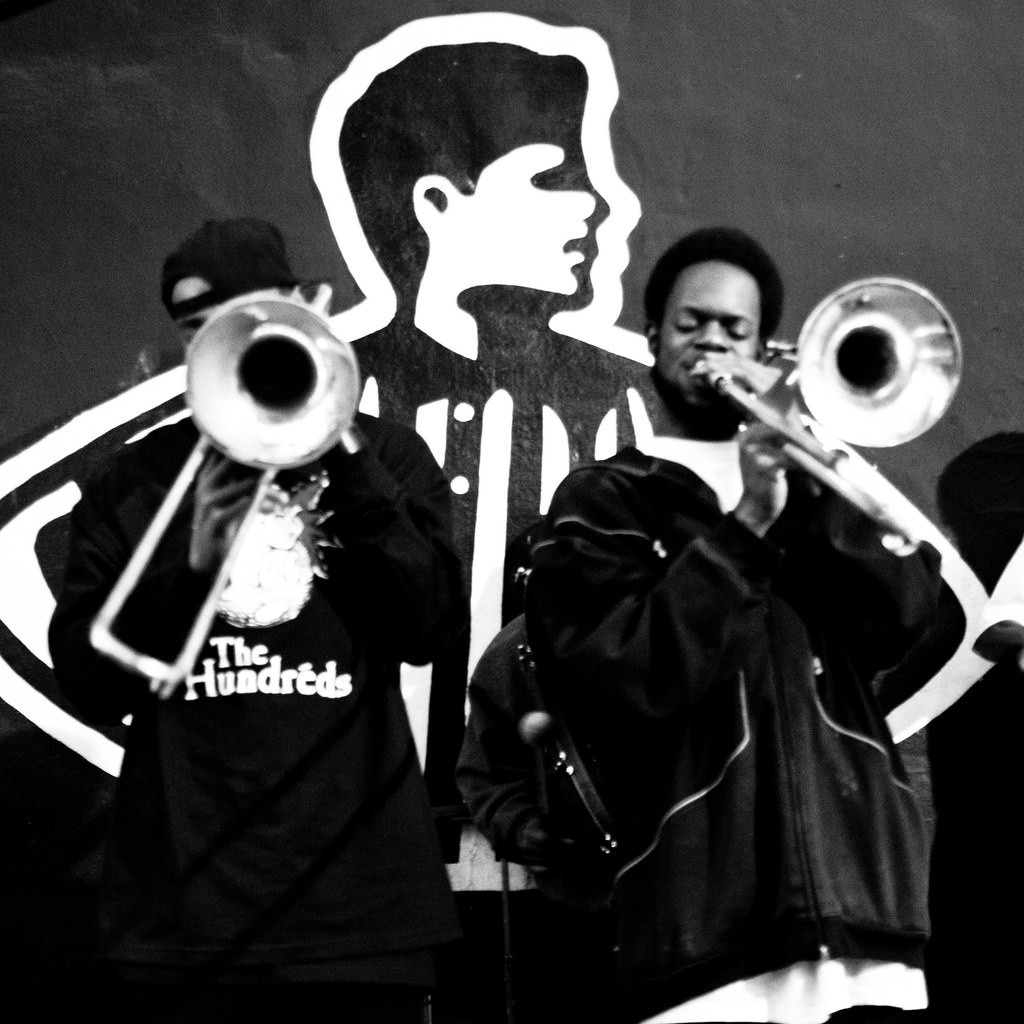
[162,217,327,321]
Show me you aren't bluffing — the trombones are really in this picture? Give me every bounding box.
[703,273,968,547]
[90,278,362,685]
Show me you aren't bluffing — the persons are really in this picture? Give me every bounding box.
[49,215,463,1024]
[531,229,938,1024]
[456,613,562,866]
